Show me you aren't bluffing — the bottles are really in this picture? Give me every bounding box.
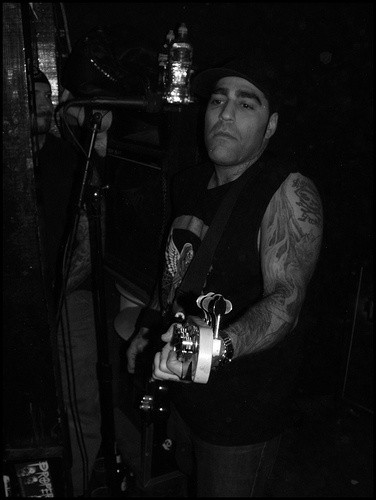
[165,26,193,103]
[158,34,176,95]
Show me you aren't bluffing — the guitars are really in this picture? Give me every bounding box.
[133,292,233,419]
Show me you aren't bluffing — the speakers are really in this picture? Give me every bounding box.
[102,140,172,295]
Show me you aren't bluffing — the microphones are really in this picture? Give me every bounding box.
[68,92,163,113]
